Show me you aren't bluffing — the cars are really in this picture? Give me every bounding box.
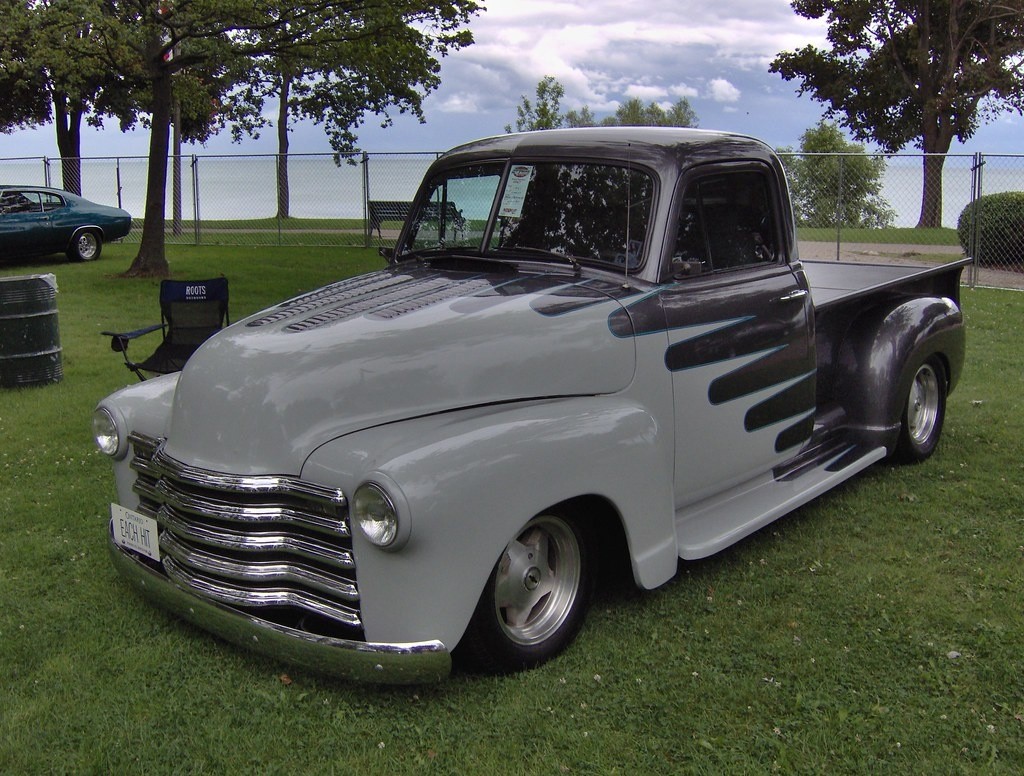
[0,184,132,265]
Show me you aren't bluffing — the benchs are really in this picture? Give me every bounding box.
[366,199,465,242]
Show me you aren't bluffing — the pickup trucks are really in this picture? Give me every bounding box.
[90,123,975,688]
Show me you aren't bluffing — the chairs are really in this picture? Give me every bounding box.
[547,200,627,262]
[100,272,231,382]
[671,202,765,272]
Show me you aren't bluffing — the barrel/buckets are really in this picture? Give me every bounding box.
[0,274,63,388]
